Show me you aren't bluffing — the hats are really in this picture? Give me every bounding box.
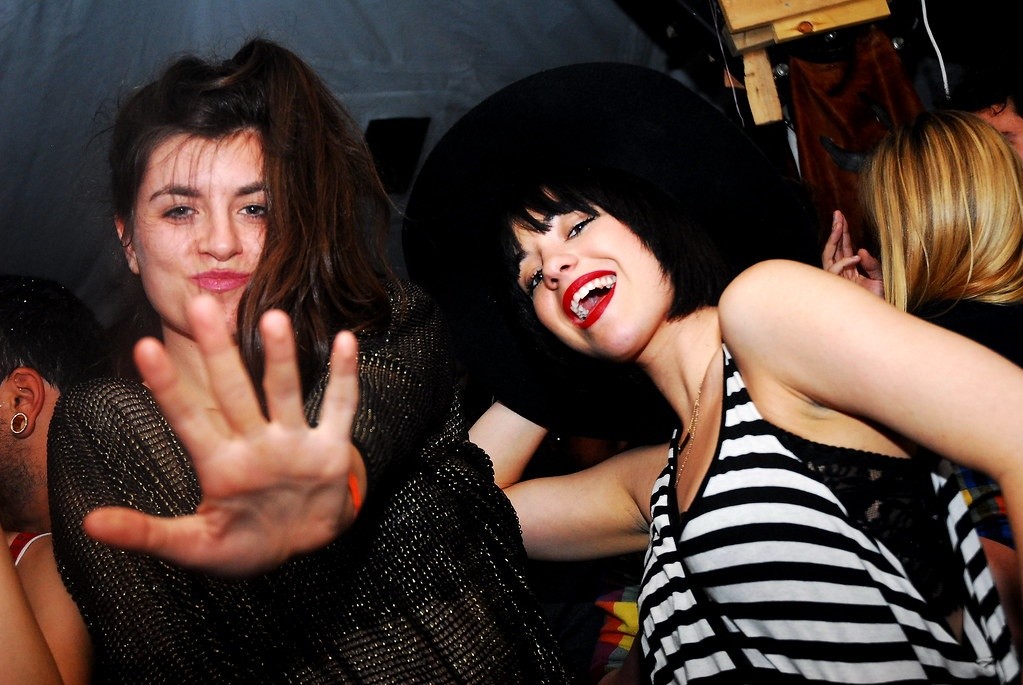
[401,63,806,441]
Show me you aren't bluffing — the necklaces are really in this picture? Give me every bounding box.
[672,386,701,489]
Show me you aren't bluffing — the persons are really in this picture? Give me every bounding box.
[0,274,109,685]
[401,50,1023,685]
[47,38,580,685]
